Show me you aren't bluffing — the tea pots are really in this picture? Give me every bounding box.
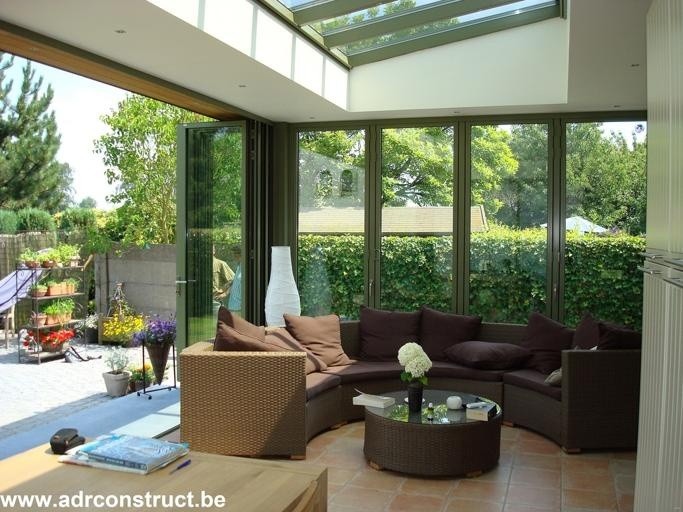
[446,396,462,410]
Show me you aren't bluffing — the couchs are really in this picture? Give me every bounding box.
[178,318,641,460]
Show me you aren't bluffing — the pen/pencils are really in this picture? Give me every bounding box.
[170,460,191,476]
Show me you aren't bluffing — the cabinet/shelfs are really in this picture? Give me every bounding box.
[14,262,86,364]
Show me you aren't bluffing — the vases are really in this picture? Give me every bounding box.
[43,341,63,352]
[408,384,423,414]
[146,342,171,385]
[130,380,150,391]
[265,246,301,325]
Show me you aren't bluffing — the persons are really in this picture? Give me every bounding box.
[214,246,242,318]
[211,244,235,316]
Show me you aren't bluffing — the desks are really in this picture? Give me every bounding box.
[0,436,328,512]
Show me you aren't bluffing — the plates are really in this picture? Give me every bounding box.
[405,397,426,404]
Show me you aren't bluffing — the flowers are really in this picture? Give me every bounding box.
[397,341,432,386]
[24,325,75,346]
[128,362,155,382]
[102,305,144,342]
[129,312,177,350]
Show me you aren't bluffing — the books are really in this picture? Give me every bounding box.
[466,402,497,422]
[352,388,396,409]
[57,433,190,475]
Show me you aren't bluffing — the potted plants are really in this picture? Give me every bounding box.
[31,297,75,325]
[19,241,84,267]
[31,277,81,297]
[104,352,130,398]
